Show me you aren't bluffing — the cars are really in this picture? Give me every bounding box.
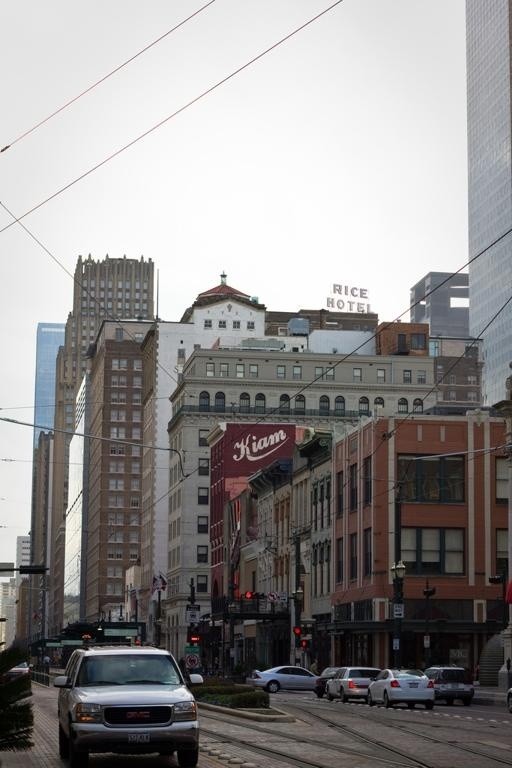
[4,662,33,681]
[506,688,512,713]
[54,645,203,768]
[254,664,474,710]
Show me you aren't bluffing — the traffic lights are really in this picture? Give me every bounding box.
[245,591,264,599]
[187,626,201,643]
[301,640,312,648]
[293,626,304,636]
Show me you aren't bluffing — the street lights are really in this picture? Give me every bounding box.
[97,611,106,642]
[390,561,405,669]
